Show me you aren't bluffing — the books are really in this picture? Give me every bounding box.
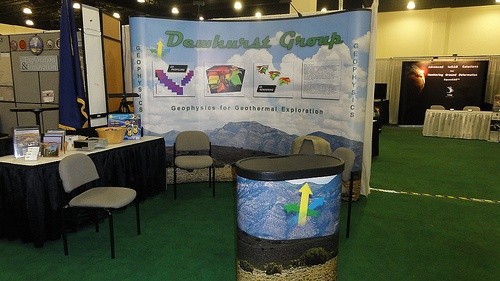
[12,127,79,162]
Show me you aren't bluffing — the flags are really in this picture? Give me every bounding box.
[58,1,90,131]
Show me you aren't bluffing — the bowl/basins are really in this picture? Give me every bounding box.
[95,127,127,143]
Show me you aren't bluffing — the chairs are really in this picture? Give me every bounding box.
[430,105,445,109]
[331,147,355,239]
[173,131,216,200]
[463,106,480,111]
[291,135,331,155]
[57,153,141,260]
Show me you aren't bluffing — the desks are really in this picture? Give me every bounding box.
[235,154,345,281]
[422,109,493,141]
[0,136,167,249]
